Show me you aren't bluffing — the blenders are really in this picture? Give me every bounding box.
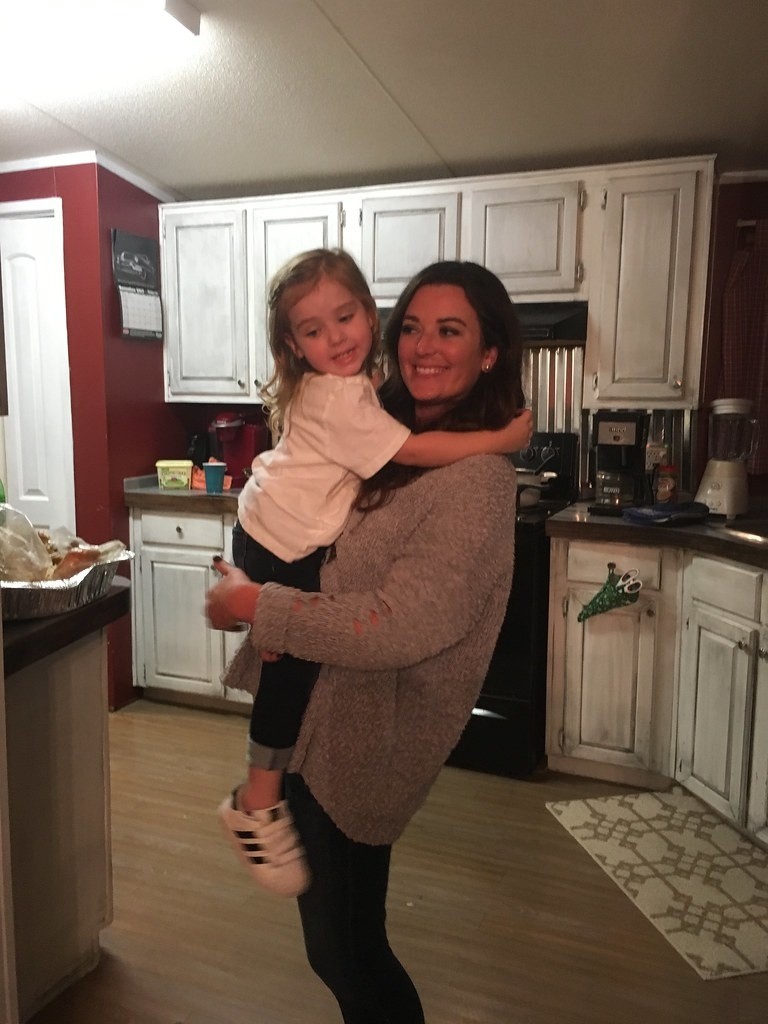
[693,397,764,520]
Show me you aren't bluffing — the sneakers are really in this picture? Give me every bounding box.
[215,785,308,896]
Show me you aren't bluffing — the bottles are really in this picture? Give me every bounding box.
[653,461,680,509]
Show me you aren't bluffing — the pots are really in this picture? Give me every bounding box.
[514,467,555,507]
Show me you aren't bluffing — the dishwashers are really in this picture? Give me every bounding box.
[444,527,549,783]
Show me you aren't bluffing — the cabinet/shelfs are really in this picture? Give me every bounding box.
[119,479,260,717]
[149,152,724,413]
[544,495,767,857]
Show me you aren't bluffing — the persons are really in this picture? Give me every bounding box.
[202,260,522,1024]
[214,243,533,897]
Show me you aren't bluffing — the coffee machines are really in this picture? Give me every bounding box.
[210,408,270,487]
[588,411,649,515]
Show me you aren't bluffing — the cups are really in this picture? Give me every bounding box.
[204,462,225,494]
[595,464,634,507]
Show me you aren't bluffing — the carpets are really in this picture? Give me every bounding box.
[544,789,767,981]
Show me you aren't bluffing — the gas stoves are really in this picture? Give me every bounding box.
[511,432,579,525]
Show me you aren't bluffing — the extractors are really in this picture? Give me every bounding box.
[363,300,589,350]
